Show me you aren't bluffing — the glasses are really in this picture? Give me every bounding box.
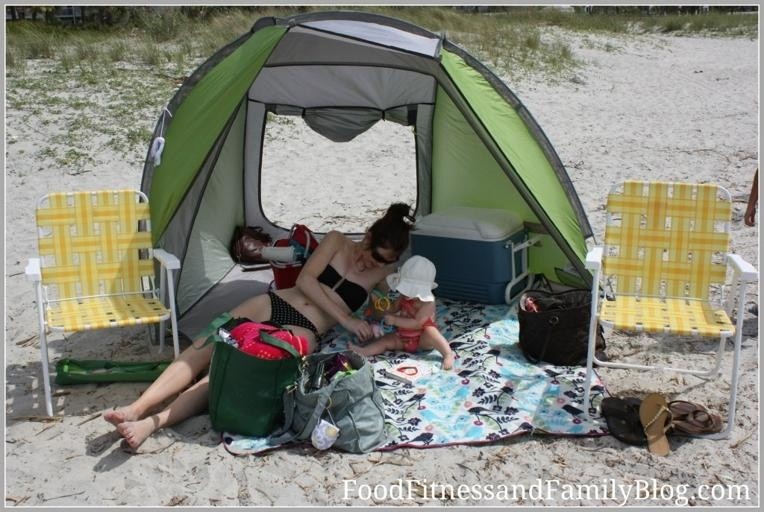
[371,249,400,265]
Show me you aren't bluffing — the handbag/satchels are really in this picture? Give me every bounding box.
[231,225,273,264]
[191,313,307,437]
[281,349,388,454]
[518,289,607,367]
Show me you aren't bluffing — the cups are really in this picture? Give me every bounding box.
[260,244,296,262]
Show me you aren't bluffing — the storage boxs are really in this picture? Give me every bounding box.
[409,207,527,305]
[270,222,319,287]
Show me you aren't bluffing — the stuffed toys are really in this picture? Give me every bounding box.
[372,288,394,334]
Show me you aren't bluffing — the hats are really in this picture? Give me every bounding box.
[386,255,438,302]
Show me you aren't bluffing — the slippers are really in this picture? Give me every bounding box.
[602,393,722,457]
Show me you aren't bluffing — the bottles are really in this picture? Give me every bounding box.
[525,297,539,312]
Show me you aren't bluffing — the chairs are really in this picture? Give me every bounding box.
[24,190,180,424]
[583,178,759,442]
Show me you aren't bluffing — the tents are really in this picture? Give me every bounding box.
[138,9,615,370]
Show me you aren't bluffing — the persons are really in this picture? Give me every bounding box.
[744,168,758,316]
[103,203,412,449]
[348,255,455,369]
